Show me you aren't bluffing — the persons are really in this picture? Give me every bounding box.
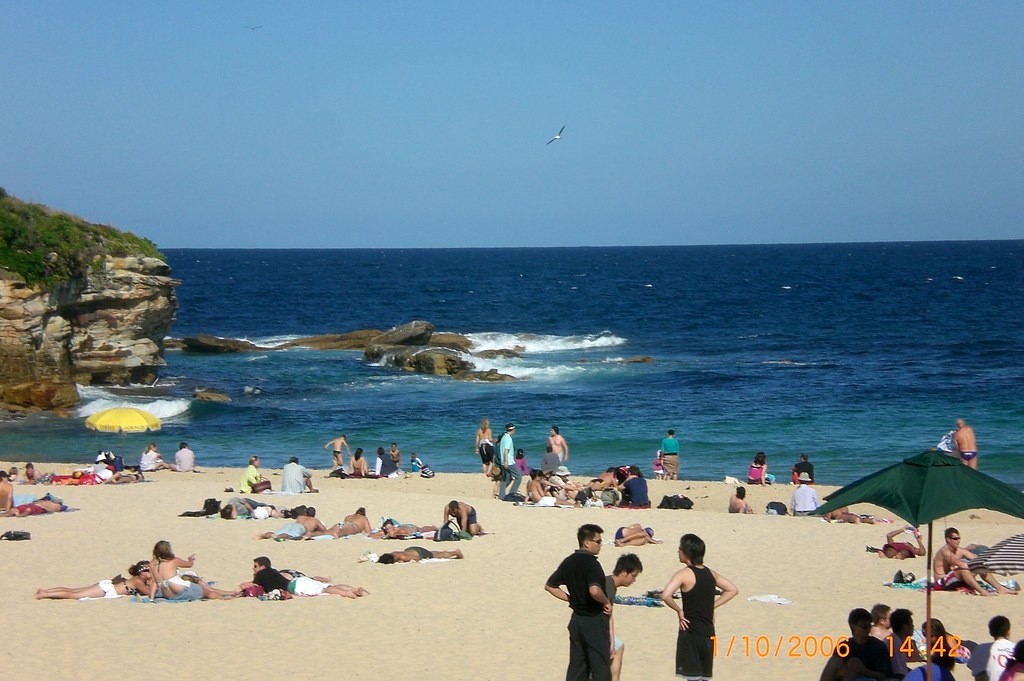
[443,501,484,535]
[934,528,1019,596]
[240,456,262,494]
[474,418,649,507]
[728,486,753,514]
[545,524,643,681]
[748,452,767,486]
[0,462,145,515]
[954,419,977,469]
[878,525,925,560]
[819,604,1024,681]
[615,524,663,547]
[140,443,169,471]
[653,429,679,479]
[36,542,369,599]
[379,547,464,564]
[222,497,438,541]
[791,472,820,515]
[170,442,194,472]
[661,534,739,681]
[324,435,422,476]
[281,457,319,494]
[826,506,874,525]
[792,453,814,484]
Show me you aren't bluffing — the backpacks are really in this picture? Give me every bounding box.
[433,521,460,542]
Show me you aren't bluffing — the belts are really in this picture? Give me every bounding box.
[797,511,809,513]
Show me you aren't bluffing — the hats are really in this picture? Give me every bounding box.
[797,472,812,481]
[556,466,571,476]
[645,528,653,537]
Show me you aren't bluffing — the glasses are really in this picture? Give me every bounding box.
[587,539,602,545]
[679,547,683,551]
[629,572,637,580]
[921,629,934,638]
[949,536,960,540]
[855,623,871,630]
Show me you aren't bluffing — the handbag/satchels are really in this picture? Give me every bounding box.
[253,477,272,492]
[245,583,265,597]
[203,498,221,515]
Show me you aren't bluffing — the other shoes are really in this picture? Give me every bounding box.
[486,471,493,477]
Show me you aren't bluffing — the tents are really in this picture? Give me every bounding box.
[967,534,1024,576]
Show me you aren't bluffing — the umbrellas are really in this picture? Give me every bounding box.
[85,407,161,435]
[817,447,1024,681]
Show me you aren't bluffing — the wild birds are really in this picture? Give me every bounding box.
[546,124,565,144]
